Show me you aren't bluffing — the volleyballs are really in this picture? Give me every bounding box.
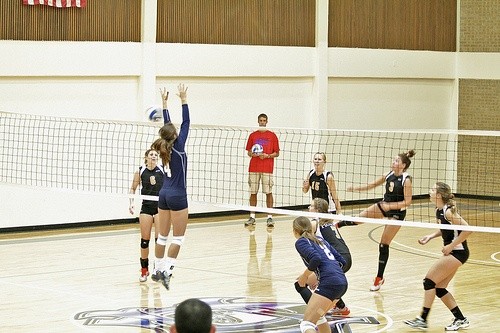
[146,107,162,122]
[252,144,263,157]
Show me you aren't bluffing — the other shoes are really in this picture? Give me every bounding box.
[152,269,172,290]
[244,216,257,226]
[139,268,149,282]
[266,218,274,227]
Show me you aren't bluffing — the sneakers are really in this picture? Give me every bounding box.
[444,316,470,331]
[370,277,384,291]
[326,304,350,315]
[403,316,428,330]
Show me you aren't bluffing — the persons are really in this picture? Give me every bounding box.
[404,182,470,330]
[294,198,352,317]
[293,216,348,333]
[335,150,417,291]
[245,114,280,226]
[152,83,190,290]
[128,149,165,282]
[170,299,215,333]
[304,152,342,215]
